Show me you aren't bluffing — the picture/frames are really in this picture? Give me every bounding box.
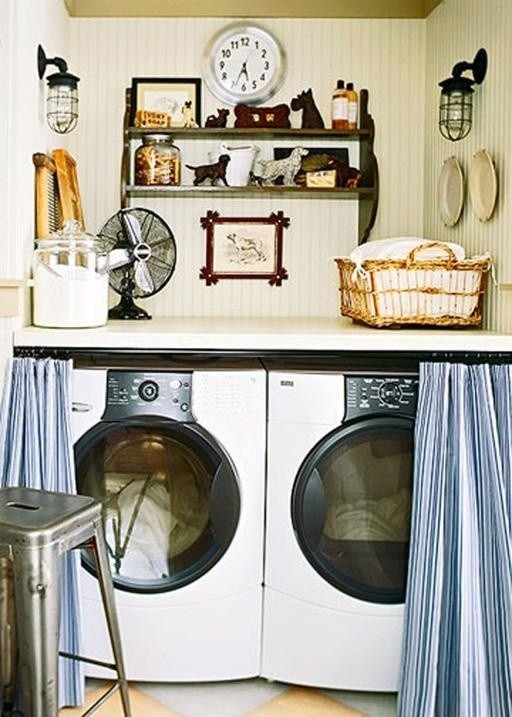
[199,209,291,289]
[128,76,204,131]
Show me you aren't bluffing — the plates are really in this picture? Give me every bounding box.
[435,156,466,228]
[466,148,498,224]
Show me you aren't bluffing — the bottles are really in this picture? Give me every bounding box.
[346,82,358,130]
[30,217,114,328]
[134,132,182,186]
[331,79,348,130]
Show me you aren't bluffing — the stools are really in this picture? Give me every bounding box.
[1,485,134,717]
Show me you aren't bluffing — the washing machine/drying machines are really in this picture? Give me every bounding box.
[259,355,420,695]
[65,357,267,683]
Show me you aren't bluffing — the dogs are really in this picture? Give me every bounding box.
[185,154,231,186]
[226,232,265,262]
[257,146,309,187]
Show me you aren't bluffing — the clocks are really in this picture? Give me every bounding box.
[199,20,290,113]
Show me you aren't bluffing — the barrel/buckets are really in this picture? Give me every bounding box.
[206,148,257,190]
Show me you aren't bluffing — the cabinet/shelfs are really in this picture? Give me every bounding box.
[120,88,380,246]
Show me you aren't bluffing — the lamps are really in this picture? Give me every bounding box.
[436,47,490,142]
[36,44,81,136]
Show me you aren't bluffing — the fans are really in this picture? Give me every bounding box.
[95,205,178,323]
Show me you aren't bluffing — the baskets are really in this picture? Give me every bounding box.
[334,241,493,329]
